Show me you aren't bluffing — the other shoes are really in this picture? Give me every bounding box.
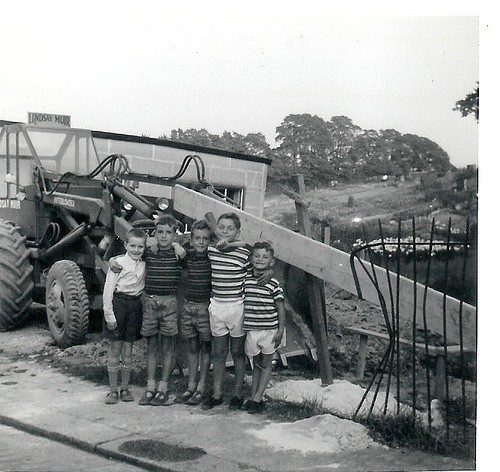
[187,390,204,405]
[149,390,169,406]
[248,400,263,414]
[228,396,244,410]
[240,398,254,411]
[119,388,134,402]
[138,389,158,405]
[201,396,223,410]
[174,388,196,403]
[105,391,119,403]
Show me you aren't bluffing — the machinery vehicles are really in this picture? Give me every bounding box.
[0,122,319,377]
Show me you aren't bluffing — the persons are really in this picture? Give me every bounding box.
[201,212,274,410]
[174,220,246,405]
[240,242,285,414]
[103,229,186,403]
[108,214,191,405]
